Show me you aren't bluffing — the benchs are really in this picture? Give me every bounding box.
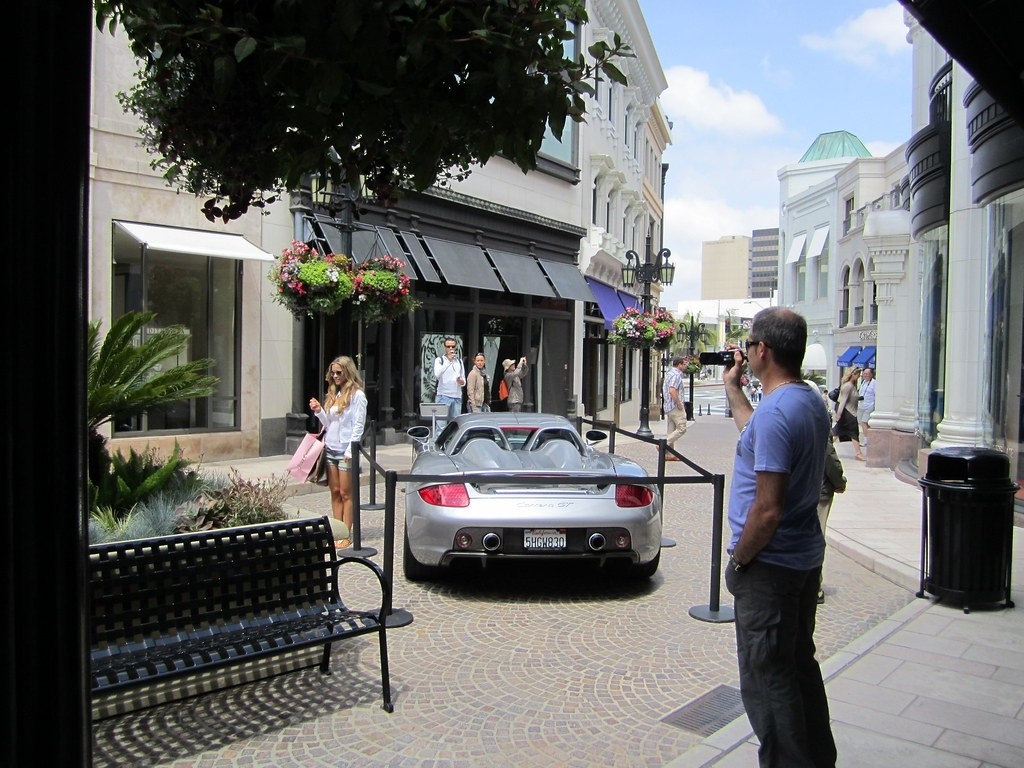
[89,514,395,713]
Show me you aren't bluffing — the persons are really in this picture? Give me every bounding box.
[722,307,838,768]
[706,368,712,380]
[309,356,368,549]
[817,438,847,604]
[750,383,762,402]
[466,354,492,413]
[830,366,868,461]
[859,368,876,447]
[656,357,687,462]
[822,390,830,412]
[433,338,465,443]
[502,356,529,412]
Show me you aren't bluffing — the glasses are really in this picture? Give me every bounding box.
[330,370,343,376]
[746,341,772,351]
[445,345,455,348]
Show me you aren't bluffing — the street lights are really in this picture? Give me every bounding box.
[676,323,707,421]
[622,249,676,439]
[662,352,674,419]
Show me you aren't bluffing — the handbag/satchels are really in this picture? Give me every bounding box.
[286,426,328,487]
[828,385,841,402]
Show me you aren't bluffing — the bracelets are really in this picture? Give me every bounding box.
[730,551,745,571]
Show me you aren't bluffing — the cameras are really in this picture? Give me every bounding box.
[699,348,744,366]
[450,351,456,360]
[858,396,864,400]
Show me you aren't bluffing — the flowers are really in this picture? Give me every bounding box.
[681,353,704,376]
[606,304,677,354]
[265,240,356,324]
[348,254,424,328]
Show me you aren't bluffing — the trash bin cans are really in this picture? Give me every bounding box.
[915,445,1019,614]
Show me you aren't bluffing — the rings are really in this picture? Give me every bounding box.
[311,402,313,405]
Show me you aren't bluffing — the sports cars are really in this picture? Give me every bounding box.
[403,410,662,583]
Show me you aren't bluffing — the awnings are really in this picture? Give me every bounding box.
[784,224,830,265]
[307,213,596,308]
[114,221,275,263]
[837,344,876,368]
[585,277,646,332]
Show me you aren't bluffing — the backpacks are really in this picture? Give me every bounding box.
[498,379,509,401]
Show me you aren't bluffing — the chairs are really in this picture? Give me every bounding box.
[465,430,496,442]
[521,429,575,449]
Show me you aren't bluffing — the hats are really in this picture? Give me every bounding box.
[502,359,516,373]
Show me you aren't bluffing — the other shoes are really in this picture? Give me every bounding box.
[860,439,868,447]
[656,445,682,461]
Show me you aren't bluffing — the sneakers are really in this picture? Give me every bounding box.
[334,538,353,550]
